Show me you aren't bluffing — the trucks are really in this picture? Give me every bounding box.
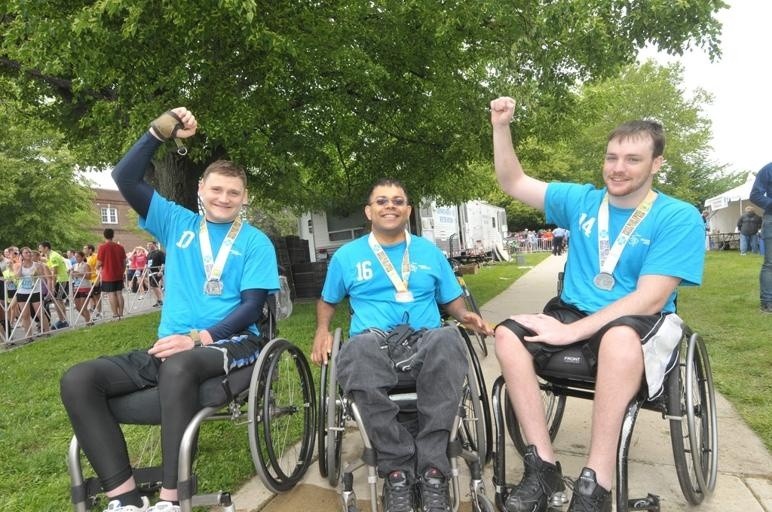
[310,195,509,264]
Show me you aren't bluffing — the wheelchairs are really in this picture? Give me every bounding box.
[68,264,316,512]
[318,277,493,512]
[493,262,716,512]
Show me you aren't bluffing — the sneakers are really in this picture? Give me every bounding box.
[382,470,416,512]
[560,467,612,512]
[505,445,565,512]
[103,496,181,512]
[10,294,164,335]
[415,468,452,512]
[760,304,772,314]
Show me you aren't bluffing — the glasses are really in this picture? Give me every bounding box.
[368,198,409,206]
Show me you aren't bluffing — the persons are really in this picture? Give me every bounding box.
[749,161,771,314]
[1,225,167,348]
[308,175,496,510]
[59,101,281,512]
[737,205,762,255]
[489,95,707,512]
[700,209,709,223]
[508,224,570,255]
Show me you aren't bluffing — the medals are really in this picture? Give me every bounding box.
[593,271,615,291]
[201,277,224,295]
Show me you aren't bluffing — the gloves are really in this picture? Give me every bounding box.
[151,110,188,156]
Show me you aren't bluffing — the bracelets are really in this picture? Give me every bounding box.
[186,328,203,347]
